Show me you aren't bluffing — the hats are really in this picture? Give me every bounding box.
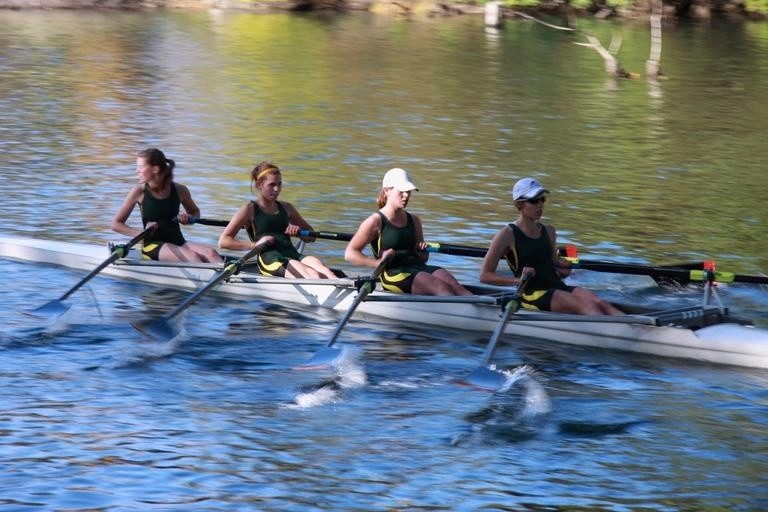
[382,168,418,192]
[512,177,550,202]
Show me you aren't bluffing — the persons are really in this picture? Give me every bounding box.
[218,162,340,283]
[344,167,473,298]
[111,148,226,268]
[479,176,630,317]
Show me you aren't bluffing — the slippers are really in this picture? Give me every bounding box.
[519,196,547,205]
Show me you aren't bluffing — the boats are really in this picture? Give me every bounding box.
[1,234,768,373]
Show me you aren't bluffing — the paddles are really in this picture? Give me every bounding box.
[425,246,716,288]
